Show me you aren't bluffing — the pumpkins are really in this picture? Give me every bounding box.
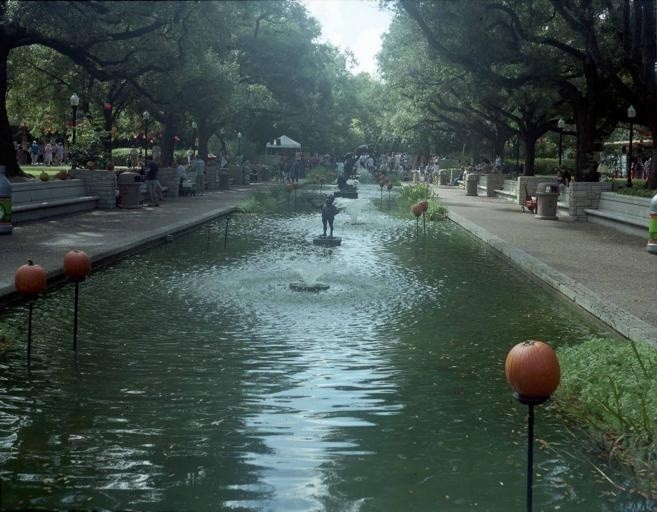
[419,202,428,211]
[504,339,562,397]
[412,205,423,215]
[64,250,91,278]
[14,260,47,289]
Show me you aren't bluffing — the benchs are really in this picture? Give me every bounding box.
[11,178,236,224]
[457,173,652,238]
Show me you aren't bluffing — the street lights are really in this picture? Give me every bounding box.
[218,128,225,154]
[556,117,566,166]
[237,132,242,155]
[624,106,638,187]
[141,110,150,165]
[192,120,198,156]
[70,91,80,167]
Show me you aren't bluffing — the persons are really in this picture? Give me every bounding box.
[630,156,650,180]
[30,140,502,210]
